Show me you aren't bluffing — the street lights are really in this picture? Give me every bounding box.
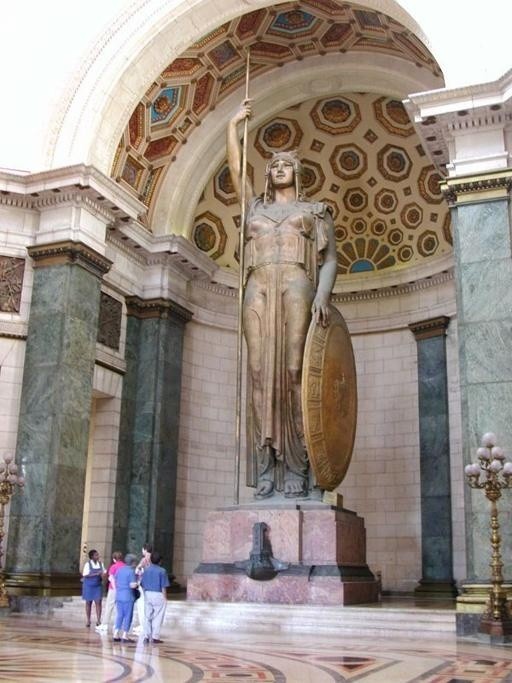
[464,431,512,636]
[0,451,25,608]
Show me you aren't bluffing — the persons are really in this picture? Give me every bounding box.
[80,541,172,644]
[224,98,339,500]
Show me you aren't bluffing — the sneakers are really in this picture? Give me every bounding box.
[86,621,163,643]
